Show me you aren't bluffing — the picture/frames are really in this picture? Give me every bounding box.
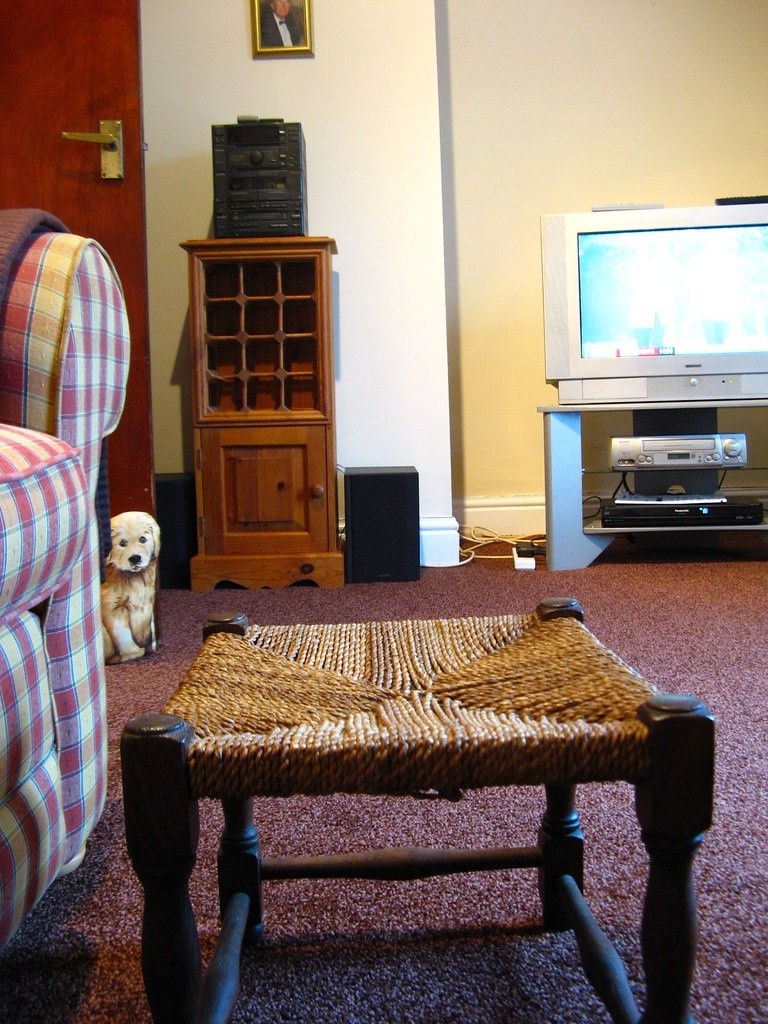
[250,0,314,62]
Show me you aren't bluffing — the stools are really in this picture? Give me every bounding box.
[122,599,718,1023]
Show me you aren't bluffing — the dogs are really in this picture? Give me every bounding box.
[97,510,162,665]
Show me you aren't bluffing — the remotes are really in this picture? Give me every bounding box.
[592,203,664,211]
[715,195,768,205]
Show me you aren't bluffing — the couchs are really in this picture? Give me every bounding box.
[1,230,132,950]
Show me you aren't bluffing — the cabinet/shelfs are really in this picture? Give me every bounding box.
[176,237,349,593]
[535,399,768,572]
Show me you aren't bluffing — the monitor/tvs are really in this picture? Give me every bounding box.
[541,203,768,406]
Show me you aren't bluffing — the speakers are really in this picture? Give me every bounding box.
[344,466,422,583]
[154,473,200,591]
